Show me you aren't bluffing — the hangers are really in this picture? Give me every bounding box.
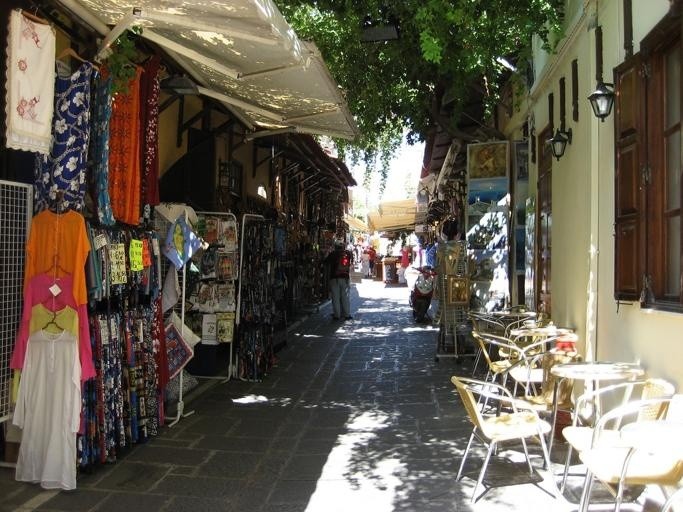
[43,254,72,342]
[46,190,71,218]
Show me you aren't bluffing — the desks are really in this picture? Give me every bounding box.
[551,359,643,428]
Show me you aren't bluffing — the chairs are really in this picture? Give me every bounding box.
[579,393,683,511]
[472,331,530,415]
[524,352,583,469]
[563,378,676,511]
[451,376,554,505]
[450,307,585,384]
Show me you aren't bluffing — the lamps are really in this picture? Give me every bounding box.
[544,83,613,161]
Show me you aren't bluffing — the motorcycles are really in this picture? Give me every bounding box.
[407,262,438,323]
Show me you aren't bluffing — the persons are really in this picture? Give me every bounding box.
[344,238,413,285]
[320,239,353,322]
